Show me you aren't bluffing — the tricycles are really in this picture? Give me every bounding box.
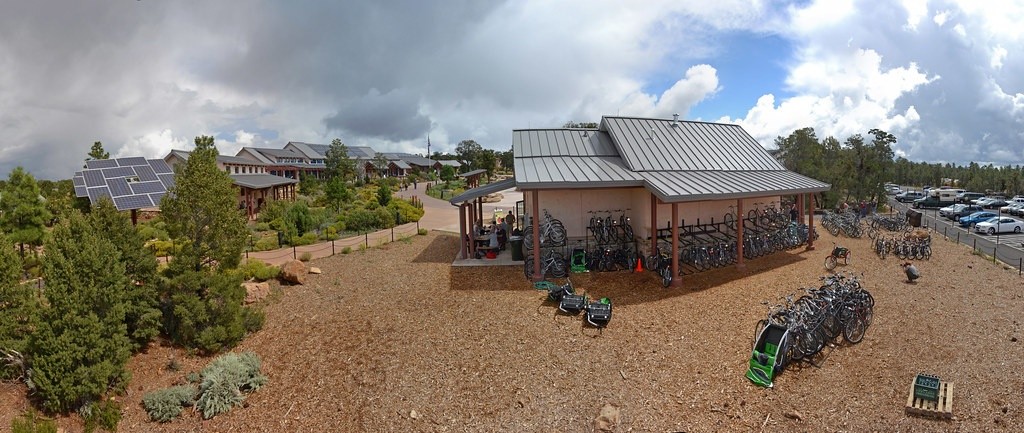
[823,240,853,271]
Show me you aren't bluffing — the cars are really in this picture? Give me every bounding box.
[878,182,1024,235]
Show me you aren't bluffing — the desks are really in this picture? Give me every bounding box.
[466,224,508,258]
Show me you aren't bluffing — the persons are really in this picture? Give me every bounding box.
[487,228,499,250]
[834,197,877,217]
[399,182,403,191]
[414,179,418,189]
[404,182,409,190]
[506,210,515,238]
[500,217,507,229]
[473,218,486,249]
[900,261,920,282]
[488,220,500,232]
[789,203,799,222]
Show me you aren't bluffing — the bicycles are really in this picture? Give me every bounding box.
[821,200,933,260]
[754,271,875,360]
[521,196,822,289]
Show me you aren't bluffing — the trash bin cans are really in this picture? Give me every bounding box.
[905,208,923,227]
[509,236,523,261]
